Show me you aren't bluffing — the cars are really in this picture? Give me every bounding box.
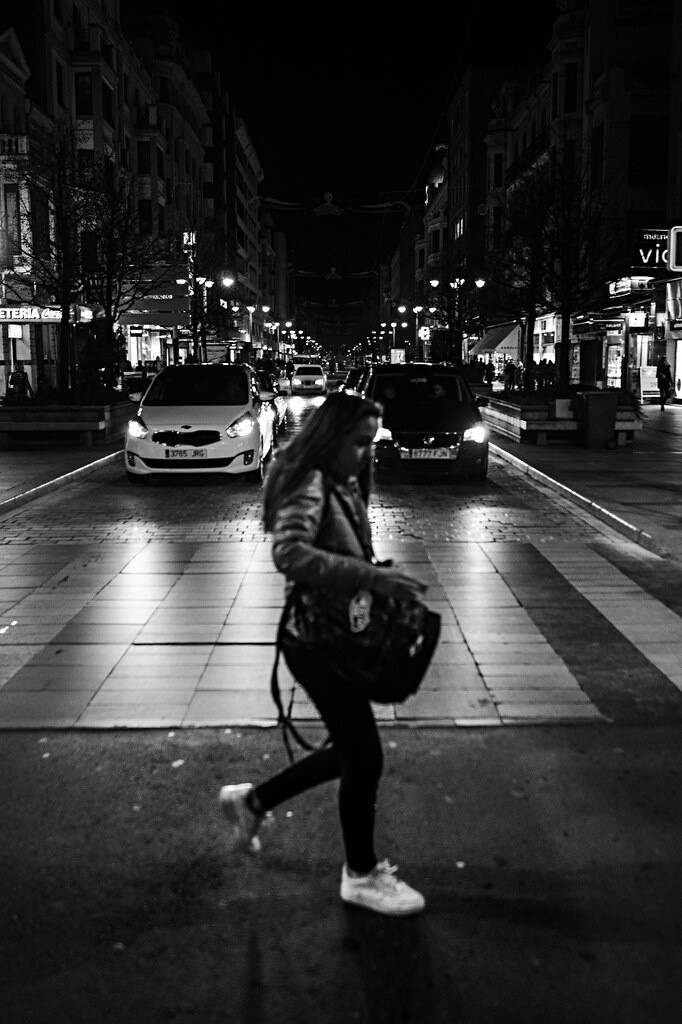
[253,351,371,394]
[124,361,274,485]
[358,364,488,485]
[254,370,289,436]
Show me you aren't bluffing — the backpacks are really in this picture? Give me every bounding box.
[271,476,443,758]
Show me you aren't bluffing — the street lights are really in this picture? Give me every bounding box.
[380,322,407,349]
[429,277,486,364]
[176,275,236,363]
[397,304,437,363]
[222,303,393,358]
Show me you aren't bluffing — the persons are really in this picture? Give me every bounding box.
[9,361,35,400]
[219,394,446,914]
[135,347,676,414]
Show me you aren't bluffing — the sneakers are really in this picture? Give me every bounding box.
[219,782,264,854]
[339,861,425,917]
